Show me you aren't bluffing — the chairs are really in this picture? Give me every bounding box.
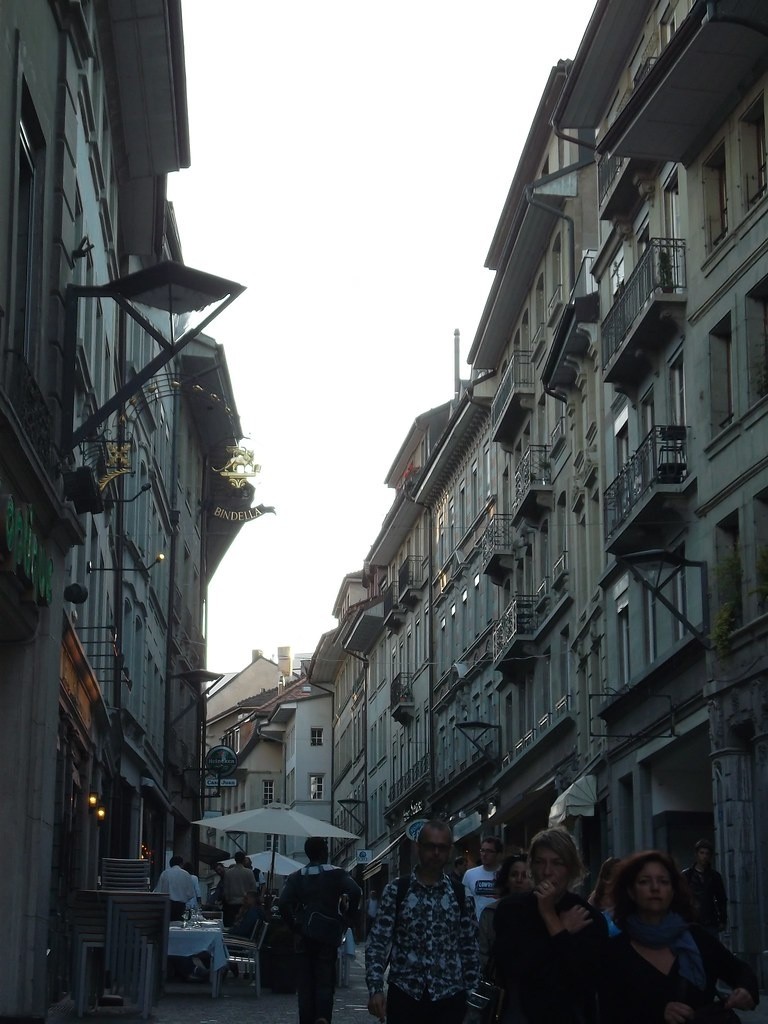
[169,885,280,1000]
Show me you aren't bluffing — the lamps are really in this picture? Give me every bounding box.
[93,667,130,681]
[96,799,106,826]
[86,554,166,573]
[74,625,117,641]
[101,481,152,504]
[81,640,120,656]
[88,783,99,814]
[97,679,132,694]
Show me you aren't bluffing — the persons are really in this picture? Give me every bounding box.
[450,833,628,975]
[152,847,271,977]
[480,826,610,1024]
[589,848,762,1024]
[676,836,730,946]
[361,820,484,1024]
[278,835,365,1023]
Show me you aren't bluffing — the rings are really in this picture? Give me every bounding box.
[543,882,550,890]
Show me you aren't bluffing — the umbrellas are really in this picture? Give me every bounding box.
[188,801,361,923]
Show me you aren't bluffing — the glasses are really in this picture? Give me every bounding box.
[480,848,498,854]
[420,842,450,852]
[508,853,527,862]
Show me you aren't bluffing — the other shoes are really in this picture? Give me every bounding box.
[315,1017,331,1024]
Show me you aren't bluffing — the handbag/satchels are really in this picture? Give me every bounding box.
[302,903,346,949]
[691,991,741,1024]
